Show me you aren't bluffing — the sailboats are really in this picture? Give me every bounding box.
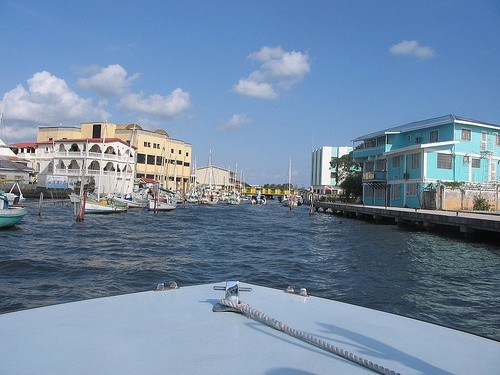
[281,156,304,207]
[66,119,269,212]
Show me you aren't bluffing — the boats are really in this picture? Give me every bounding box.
[1,179,27,207]
[0,188,27,228]
